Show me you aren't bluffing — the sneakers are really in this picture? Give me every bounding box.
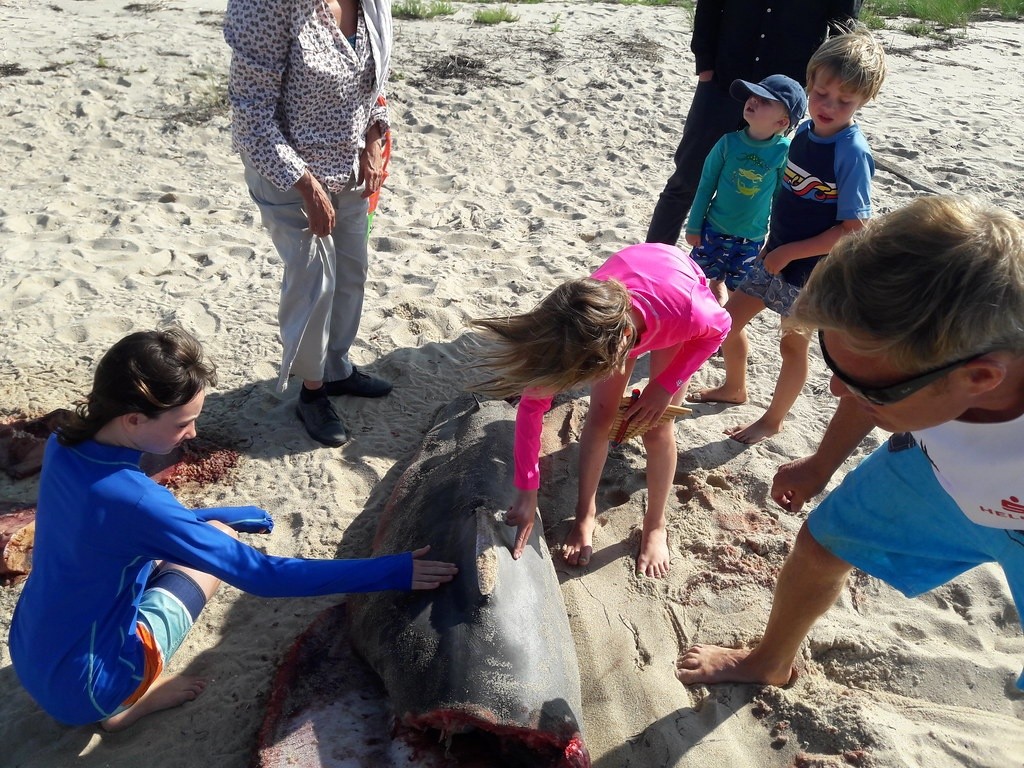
[325,366,394,398]
[296,392,347,446]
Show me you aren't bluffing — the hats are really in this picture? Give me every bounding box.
[730,75,807,128]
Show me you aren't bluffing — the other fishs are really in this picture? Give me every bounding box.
[251,390,593,768]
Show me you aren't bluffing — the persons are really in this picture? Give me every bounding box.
[8,327,458,733]
[684,73,808,306]
[222,0,392,446]
[674,195,1024,696]
[470,243,732,581]
[685,32,885,444]
[647,0,857,245]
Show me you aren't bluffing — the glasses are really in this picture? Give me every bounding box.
[818,328,1015,406]
